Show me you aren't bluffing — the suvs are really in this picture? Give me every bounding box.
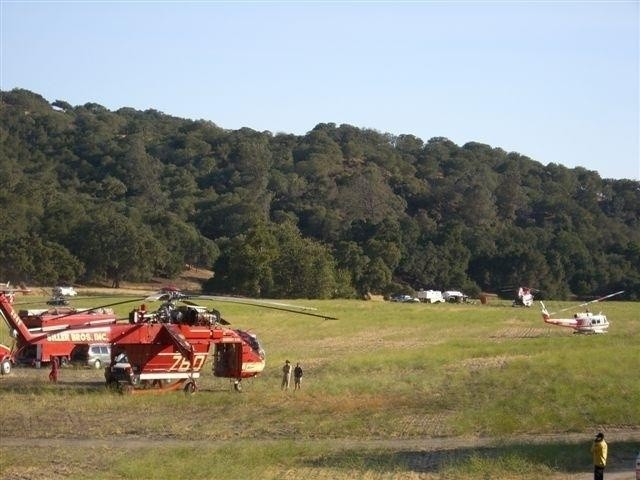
[0,287,341,398]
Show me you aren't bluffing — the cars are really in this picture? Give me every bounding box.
[392,295,420,304]
[1,342,17,376]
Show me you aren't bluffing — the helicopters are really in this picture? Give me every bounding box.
[536,290,625,335]
[497,284,548,308]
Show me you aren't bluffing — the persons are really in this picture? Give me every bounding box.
[49,359,59,384]
[294,362,304,390]
[281,360,292,389]
[590,433,609,480]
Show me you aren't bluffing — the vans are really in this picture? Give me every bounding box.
[70,343,112,370]
[57,286,78,297]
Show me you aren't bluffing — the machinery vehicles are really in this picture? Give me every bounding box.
[1,289,117,367]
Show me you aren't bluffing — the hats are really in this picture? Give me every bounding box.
[595,433,604,438]
[286,360,290,363]
